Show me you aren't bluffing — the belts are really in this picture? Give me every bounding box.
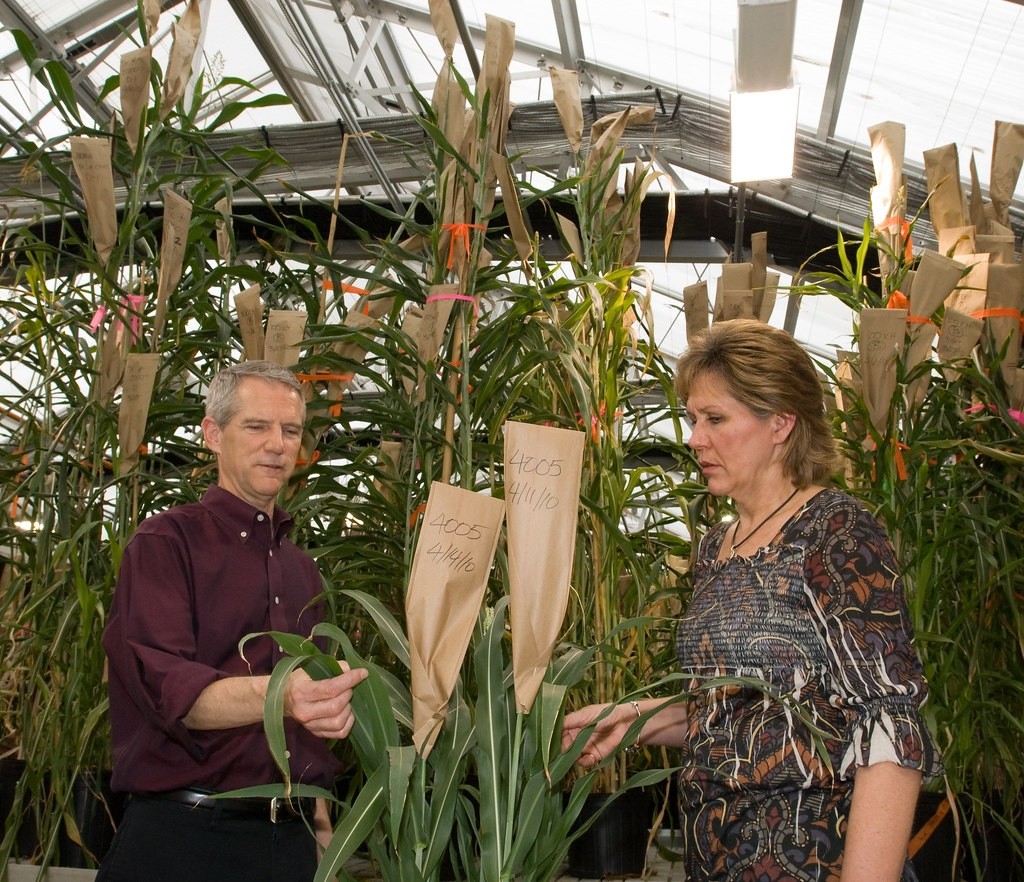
[175,784,314,826]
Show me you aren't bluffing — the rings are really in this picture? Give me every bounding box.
[590,754,596,759]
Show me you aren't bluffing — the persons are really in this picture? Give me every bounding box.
[92,359,370,882]
[556,316,949,881]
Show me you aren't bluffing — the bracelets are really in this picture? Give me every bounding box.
[622,700,642,753]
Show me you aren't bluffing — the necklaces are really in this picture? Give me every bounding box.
[728,487,801,559]
